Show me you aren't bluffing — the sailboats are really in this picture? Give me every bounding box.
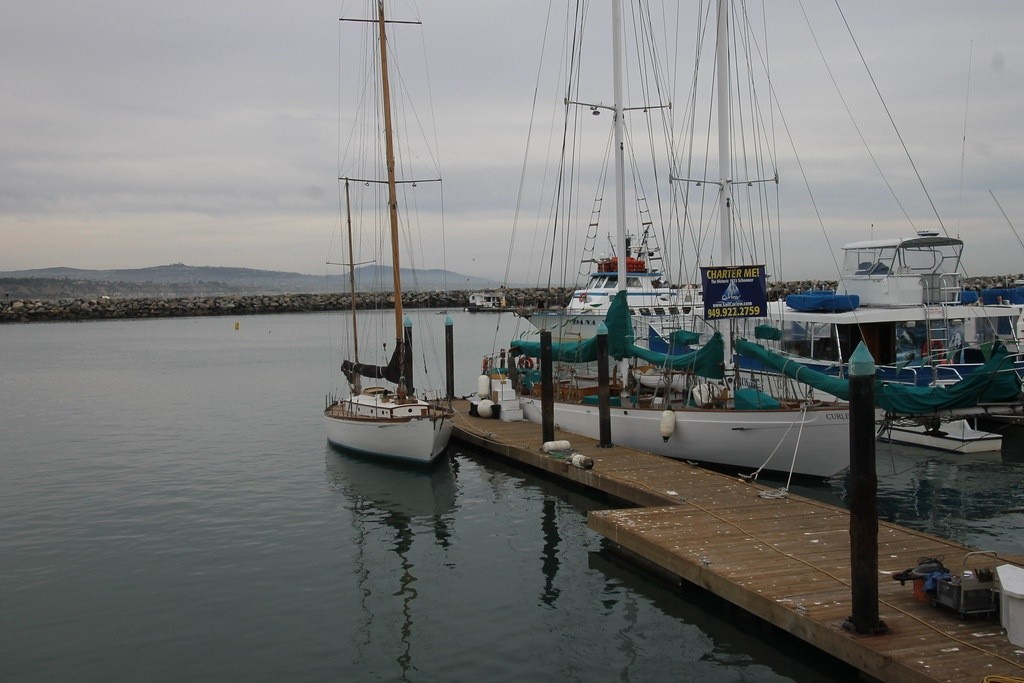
[322,0,457,466]
[465,1,1024,479]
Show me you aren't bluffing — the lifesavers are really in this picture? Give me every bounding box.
[921,338,943,359]
[579,293,587,302]
[518,357,533,369]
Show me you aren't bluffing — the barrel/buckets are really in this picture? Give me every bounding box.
[911,564,949,600]
